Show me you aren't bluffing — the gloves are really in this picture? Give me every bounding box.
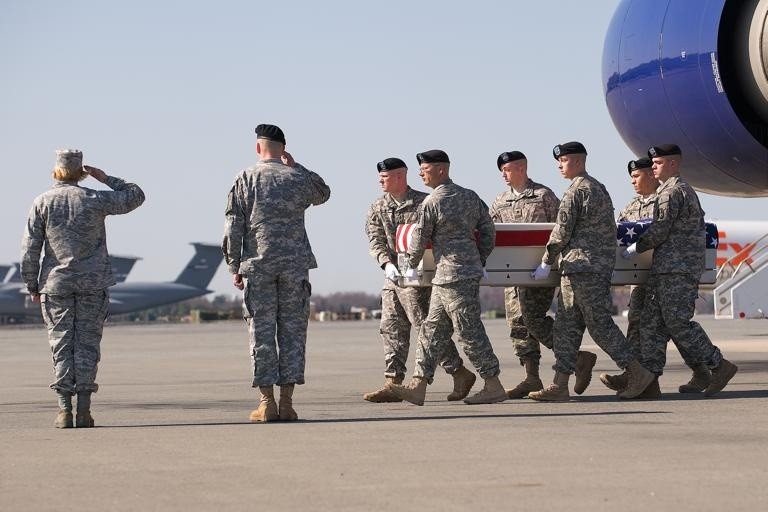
[481,267,488,281]
[621,242,640,259]
[530,261,552,280]
[403,267,420,281]
[384,263,402,282]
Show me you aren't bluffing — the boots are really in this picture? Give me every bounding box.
[506,351,597,402]
[249,385,277,422]
[463,376,506,404]
[679,359,737,397]
[447,365,476,401]
[279,383,298,421]
[76,391,94,427]
[362,376,428,405]
[599,359,662,400]
[54,392,73,428]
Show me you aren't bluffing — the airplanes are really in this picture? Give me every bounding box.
[0,242,227,324]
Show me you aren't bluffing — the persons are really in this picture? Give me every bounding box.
[526,142,657,401]
[20,149,145,428]
[384,149,509,405]
[221,124,330,422]
[486,150,597,399]
[600,157,712,394]
[362,158,477,403]
[615,142,738,400]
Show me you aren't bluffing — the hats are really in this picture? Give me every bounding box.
[416,150,449,166]
[55,149,82,169]
[378,158,407,172]
[255,124,285,145]
[628,158,653,175]
[647,144,682,160]
[553,141,587,161]
[497,151,526,171]
